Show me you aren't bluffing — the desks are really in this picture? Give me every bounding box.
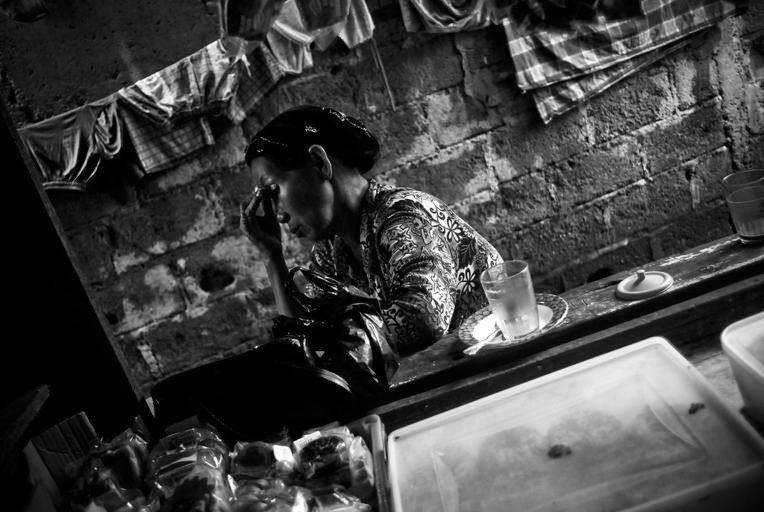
[277,226,764,422]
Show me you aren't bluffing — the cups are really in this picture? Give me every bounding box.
[478,260,539,334]
[722,170,764,245]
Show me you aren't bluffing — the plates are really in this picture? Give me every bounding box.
[457,292,570,350]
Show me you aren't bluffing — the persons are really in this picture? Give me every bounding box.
[237,103,512,358]
